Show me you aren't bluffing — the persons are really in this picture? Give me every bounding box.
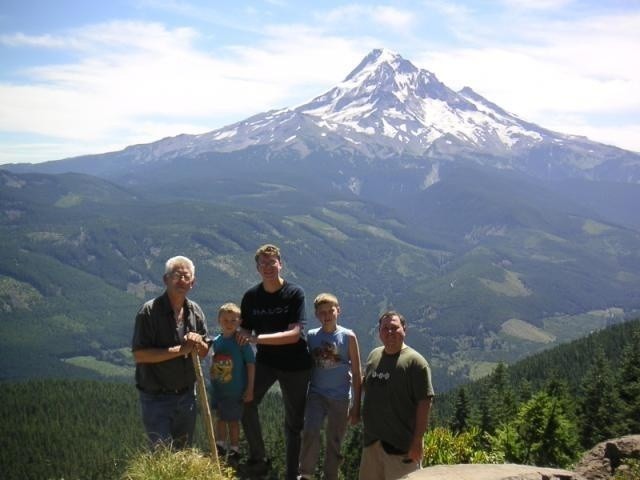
[131,256,209,457]
[236,244,311,480]
[207,302,259,461]
[293,292,362,480]
[358,310,435,480]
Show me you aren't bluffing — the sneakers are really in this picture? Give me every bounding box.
[238,458,272,476]
[217,444,240,458]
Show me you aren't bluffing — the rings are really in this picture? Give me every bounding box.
[246,337,249,342]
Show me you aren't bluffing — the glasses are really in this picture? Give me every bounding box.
[402,457,413,465]
[257,260,279,267]
[168,272,193,279]
